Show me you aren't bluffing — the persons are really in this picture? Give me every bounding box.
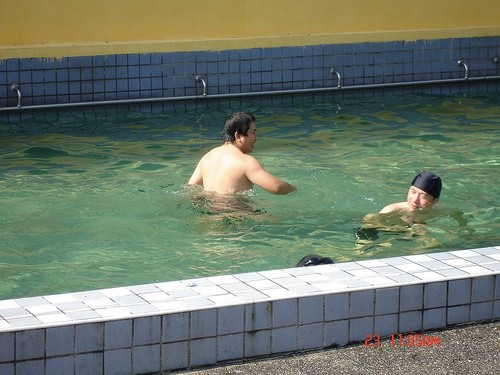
[295,253,335,268]
[183,112,298,195]
[363,169,442,218]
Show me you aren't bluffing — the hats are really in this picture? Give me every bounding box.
[296,254,334,267]
[411,171,443,198]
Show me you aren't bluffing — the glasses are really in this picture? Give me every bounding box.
[303,257,333,266]
